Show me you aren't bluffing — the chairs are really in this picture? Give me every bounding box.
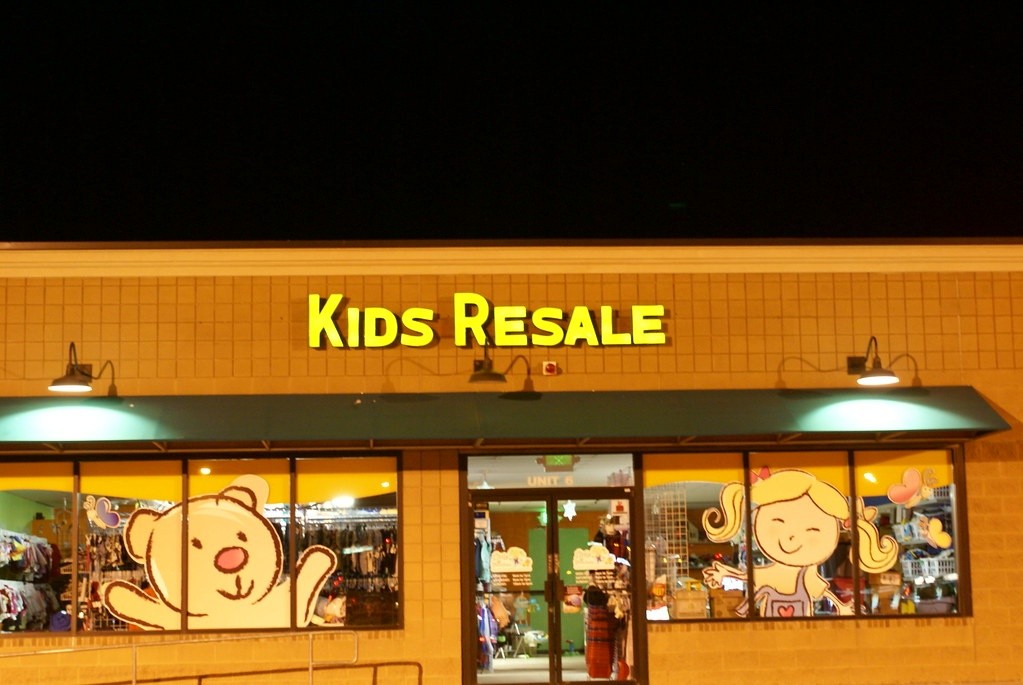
[495,615,528,660]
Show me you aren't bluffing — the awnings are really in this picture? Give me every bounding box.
[0,387,1012,463]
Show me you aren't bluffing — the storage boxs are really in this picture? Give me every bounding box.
[668,591,708,619]
[868,572,901,586]
[929,486,949,499]
[900,559,922,578]
[919,557,955,576]
[892,523,923,543]
[710,589,744,619]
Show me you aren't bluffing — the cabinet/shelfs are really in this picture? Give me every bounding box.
[655,538,712,573]
[903,499,957,595]
[59,534,96,631]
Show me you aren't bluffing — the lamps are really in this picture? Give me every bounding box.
[48,342,93,392]
[468,337,507,384]
[848,335,899,386]
